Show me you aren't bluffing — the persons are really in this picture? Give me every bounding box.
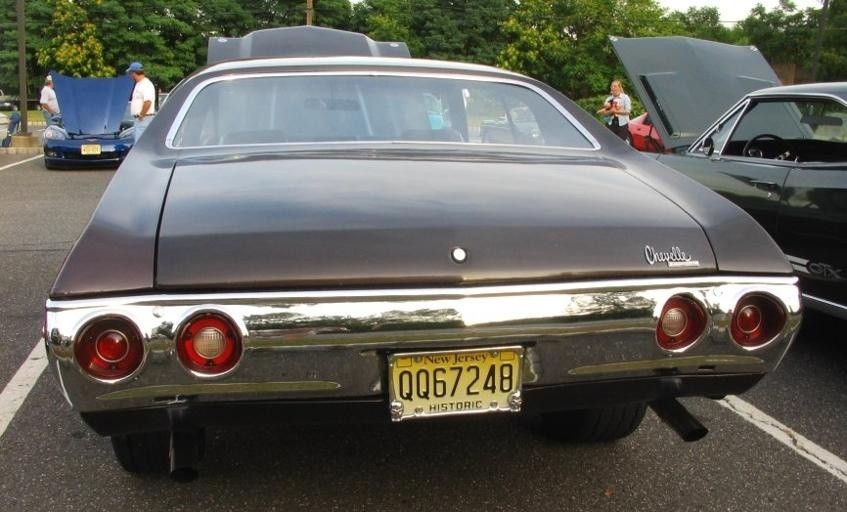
[153,80,162,113]
[462,87,470,109]
[605,81,631,145]
[2,105,21,146]
[39,74,60,125]
[126,59,155,144]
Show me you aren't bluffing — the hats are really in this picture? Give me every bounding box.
[126,62,143,72]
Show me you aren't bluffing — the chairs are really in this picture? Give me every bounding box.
[402,128,465,141]
[220,130,287,144]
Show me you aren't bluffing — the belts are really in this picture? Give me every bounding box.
[134,114,154,118]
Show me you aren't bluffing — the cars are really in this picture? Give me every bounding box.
[40,69,138,170]
[607,38,846,320]
[41,24,805,477]
[624,110,669,154]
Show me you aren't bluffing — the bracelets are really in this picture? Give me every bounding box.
[139,114,143,118]
